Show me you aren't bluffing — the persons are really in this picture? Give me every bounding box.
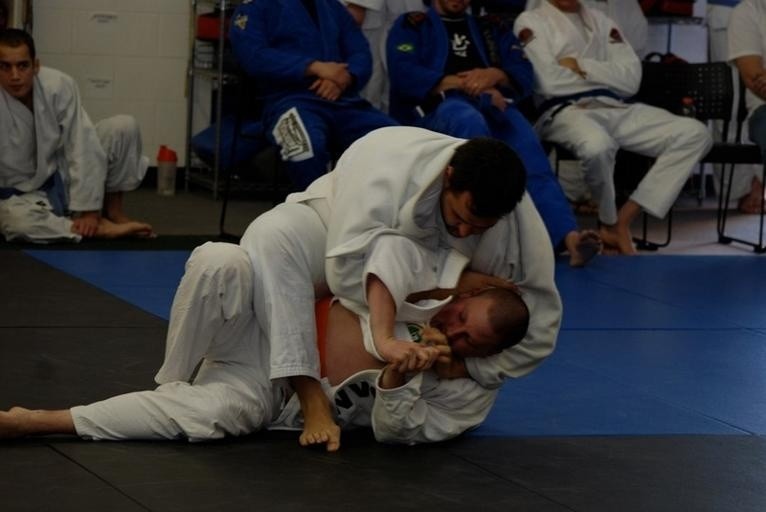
[236,124,565,453]
[0,27,158,245]
[0,234,529,444]
[226,0,712,271]
[727,2,765,141]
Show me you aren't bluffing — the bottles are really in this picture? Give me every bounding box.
[157,145,179,196]
[683,97,695,118]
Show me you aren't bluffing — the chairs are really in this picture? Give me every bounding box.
[186,62,766,254]
